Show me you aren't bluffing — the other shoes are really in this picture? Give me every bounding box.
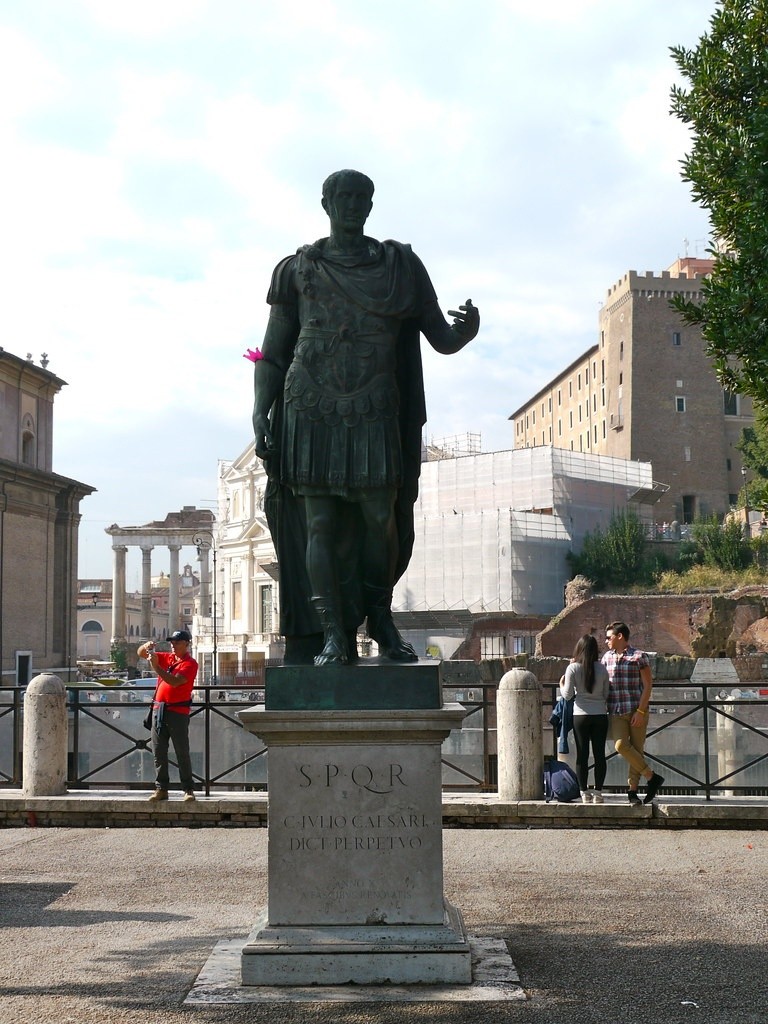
[643,770,665,804]
[626,790,642,805]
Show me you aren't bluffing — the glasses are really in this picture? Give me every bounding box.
[605,636,611,639]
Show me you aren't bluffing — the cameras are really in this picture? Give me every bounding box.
[147,648,155,657]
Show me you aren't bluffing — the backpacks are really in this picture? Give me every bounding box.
[544,755,581,803]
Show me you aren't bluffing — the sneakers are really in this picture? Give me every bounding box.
[184,789,195,801]
[580,789,593,803]
[593,790,604,804]
[149,788,168,801]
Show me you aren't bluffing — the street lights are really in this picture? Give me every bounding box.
[741,466,752,534]
[192,530,217,685]
[672,505,678,521]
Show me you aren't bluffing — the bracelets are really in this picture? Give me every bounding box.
[637,709,644,714]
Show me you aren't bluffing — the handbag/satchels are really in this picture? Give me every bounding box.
[143,708,153,731]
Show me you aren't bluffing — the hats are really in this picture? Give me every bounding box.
[166,630,190,642]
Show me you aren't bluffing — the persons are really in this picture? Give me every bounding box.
[76,668,113,683]
[663,521,668,533]
[254,169,482,665]
[601,621,665,807]
[560,634,609,804]
[137,630,199,802]
[143,672,152,678]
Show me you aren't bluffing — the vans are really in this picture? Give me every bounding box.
[64,682,116,703]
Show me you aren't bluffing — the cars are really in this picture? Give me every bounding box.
[119,678,159,702]
[93,678,130,687]
